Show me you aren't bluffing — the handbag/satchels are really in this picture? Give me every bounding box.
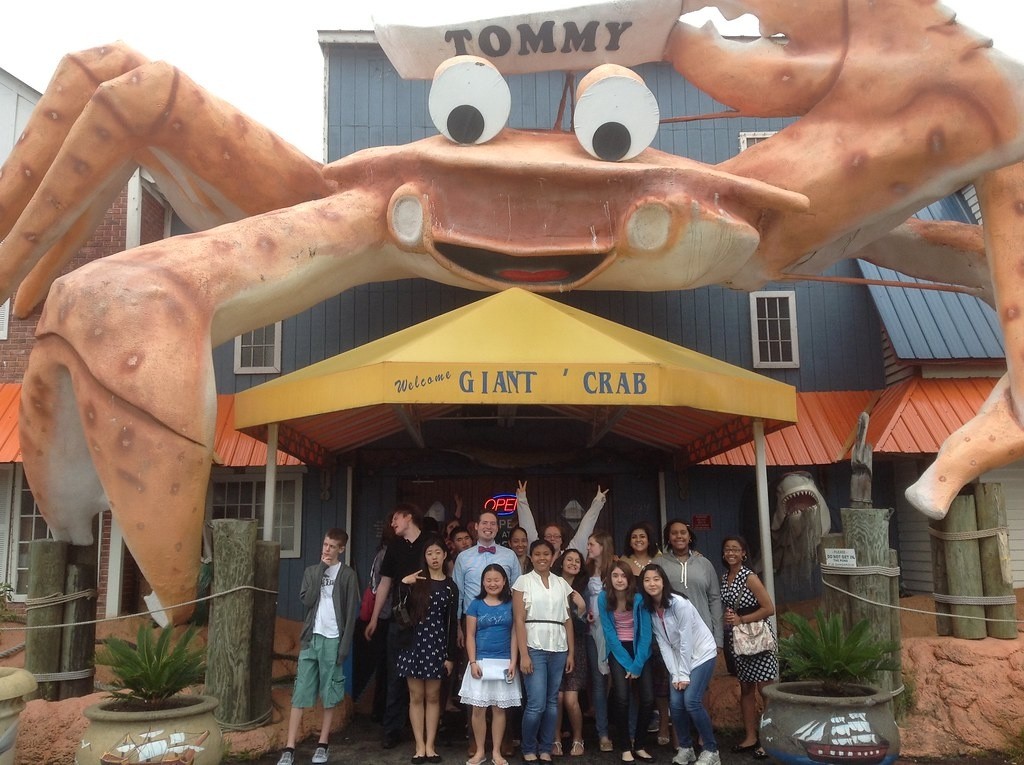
[360,583,376,622]
[732,621,775,656]
[392,602,412,631]
[446,644,466,664]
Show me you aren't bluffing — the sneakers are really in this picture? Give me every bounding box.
[672,747,696,765]
[312,745,330,763]
[694,749,721,765]
[276,750,295,765]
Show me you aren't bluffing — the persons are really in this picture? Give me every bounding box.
[347,530,388,726]
[619,525,663,583]
[457,564,523,764]
[569,530,614,752]
[511,539,573,763]
[596,560,652,763]
[636,564,721,765]
[649,520,722,749]
[278,530,361,761]
[550,544,593,756]
[362,495,480,616]
[516,480,610,567]
[365,505,455,748]
[717,535,779,757]
[507,526,534,574]
[397,538,461,765]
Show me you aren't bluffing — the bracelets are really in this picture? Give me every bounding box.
[469,660,477,665]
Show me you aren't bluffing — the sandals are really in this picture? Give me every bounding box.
[570,739,584,757]
[464,752,487,765]
[491,753,508,765]
[550,742,565,757]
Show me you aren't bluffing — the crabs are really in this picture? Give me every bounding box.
[0,0,1024,631]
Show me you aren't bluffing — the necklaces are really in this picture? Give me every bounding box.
[632,557,652,571]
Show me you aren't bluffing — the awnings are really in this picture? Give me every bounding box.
[0,382,309,473]
[229,281,797,706]
[679,375,1020,467]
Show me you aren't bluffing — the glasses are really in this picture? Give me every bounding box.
[723,547,743,553]
[543,534,562,539]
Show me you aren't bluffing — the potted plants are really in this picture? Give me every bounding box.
[72,616,225,763]
[755,602,905,762]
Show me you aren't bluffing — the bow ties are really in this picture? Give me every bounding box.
[478,545,496,554]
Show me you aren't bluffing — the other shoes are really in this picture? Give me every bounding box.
[599,739,614,751]
[381,728,400,748]
[731,739,761,753]
[633,750,656,763]
[538,753,553,765]
[751,745,769,759]
[648,709,674,733]
[521,753,540,765]
[466,736,478,757]
[621,753,638,765]
[658,734,670,745]
[411,751,426,764]
[425,752,442,764]
[499,739,516,757]
